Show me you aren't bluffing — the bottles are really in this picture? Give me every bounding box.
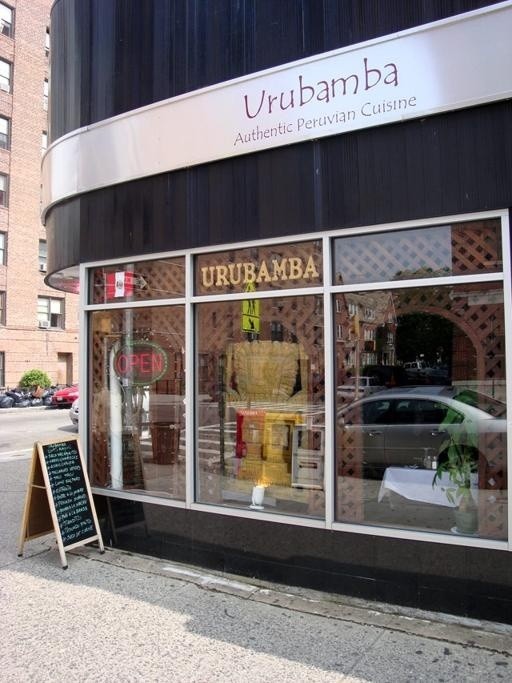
[425,456,438,469]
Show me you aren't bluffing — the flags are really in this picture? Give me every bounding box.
[104,272,133,298]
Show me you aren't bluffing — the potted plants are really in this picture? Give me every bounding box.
[433,382,498,535]
[19,368,53,398]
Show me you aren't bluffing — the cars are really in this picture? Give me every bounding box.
[52,385,150,432]
[335,384,507,489]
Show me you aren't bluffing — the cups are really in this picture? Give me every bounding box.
[252,486,265,505]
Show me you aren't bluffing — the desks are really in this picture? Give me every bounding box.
[379,465,480,521]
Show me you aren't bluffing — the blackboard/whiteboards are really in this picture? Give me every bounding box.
[108,432,145,530]
[36,436,100,552]
